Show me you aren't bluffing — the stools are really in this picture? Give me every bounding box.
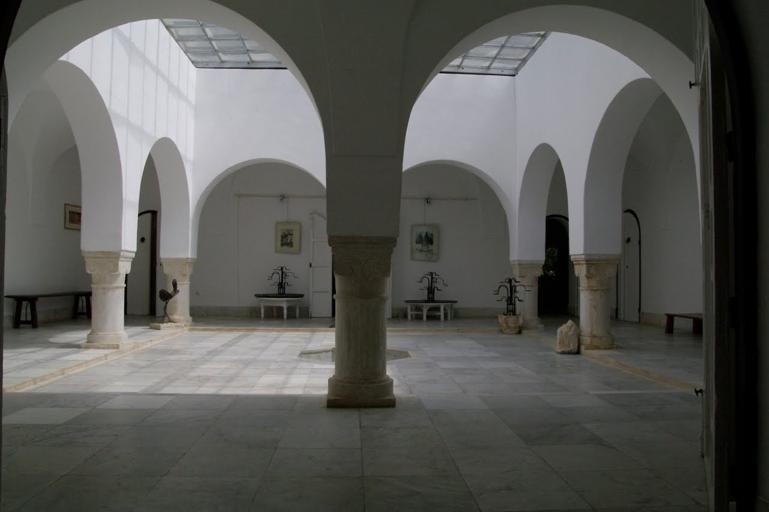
[254,294,305,319]
[404,299,458,321]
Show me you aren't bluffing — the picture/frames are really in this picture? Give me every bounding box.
[274,221,301,253]
[411,224,440,263]
[64,203,81,230]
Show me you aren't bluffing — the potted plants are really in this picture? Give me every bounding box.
[267,264,299,293]
[417,271,449,299]
[493,276,526,335]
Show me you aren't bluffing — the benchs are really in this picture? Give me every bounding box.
[664,313,703,335]
[4,291,92,329]
